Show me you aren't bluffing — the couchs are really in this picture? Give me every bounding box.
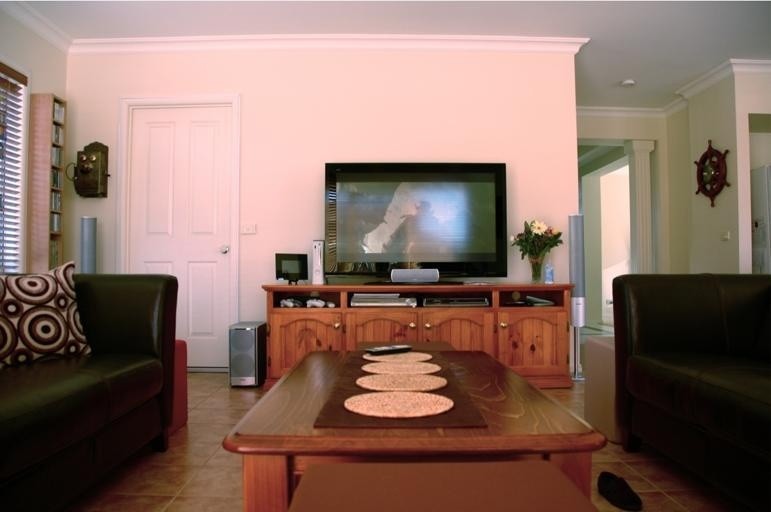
[0,273,179,511]
[612,272,771,512]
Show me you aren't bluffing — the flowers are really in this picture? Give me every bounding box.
[510,219,565,280]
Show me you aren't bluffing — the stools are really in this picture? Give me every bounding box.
[288,459,600,511]
[582,335,623,443]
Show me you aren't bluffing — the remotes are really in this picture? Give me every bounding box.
[366,344,413,356]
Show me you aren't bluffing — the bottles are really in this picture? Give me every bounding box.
[544,258,554,284]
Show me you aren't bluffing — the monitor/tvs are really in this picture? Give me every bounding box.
[324,162,507,285]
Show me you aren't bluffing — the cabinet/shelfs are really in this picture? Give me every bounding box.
[26,91,69,277]
[261,282,575,391]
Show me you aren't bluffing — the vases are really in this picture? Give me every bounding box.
[529,255,545,284]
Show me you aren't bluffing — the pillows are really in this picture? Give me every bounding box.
[0,260,93,370]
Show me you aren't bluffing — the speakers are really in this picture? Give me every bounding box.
[228,320,267,387]
[568,214,586,381]
[391,268,440,283]
[79,216,98,274]
